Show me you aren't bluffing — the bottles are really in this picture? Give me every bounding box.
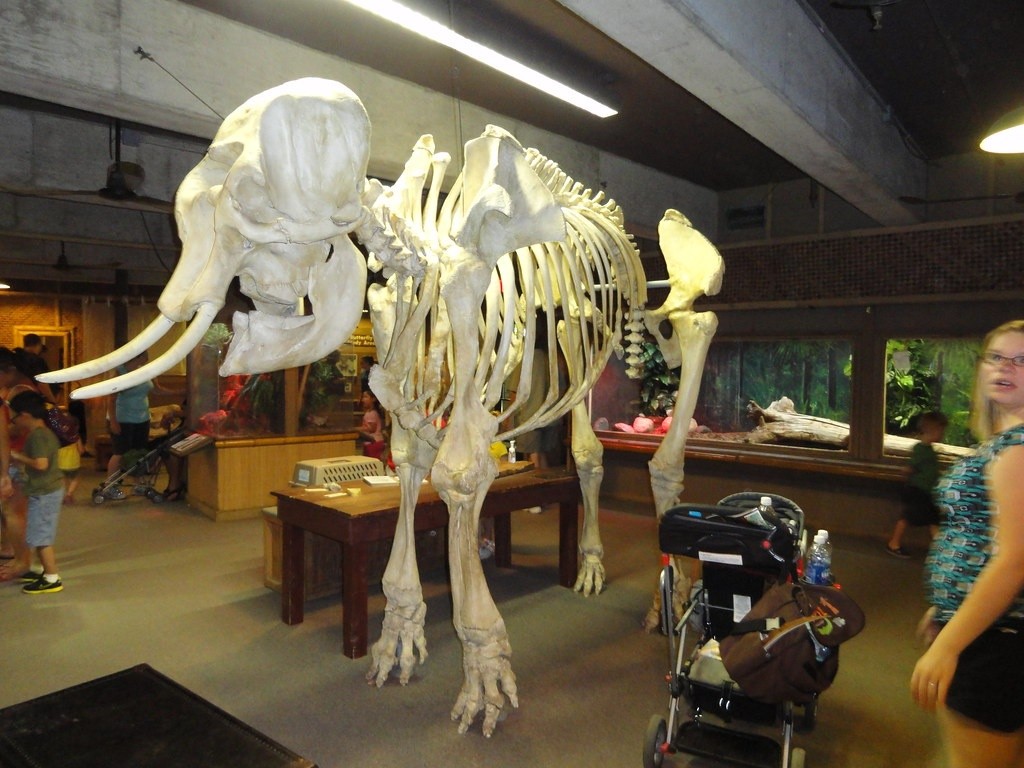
[758,496,776,530]
[818,529,831,563]
[804,535,832,586]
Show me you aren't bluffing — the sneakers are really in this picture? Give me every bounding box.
[23,572,63,594]
[19,570,45,582]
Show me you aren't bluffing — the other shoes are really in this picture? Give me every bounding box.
[0,551,15,560]
[63,495,74,506]
[108,488,127,500]
[886,544,910,558]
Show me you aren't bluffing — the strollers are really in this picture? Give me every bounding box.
[92,427,185,504]
[642,490,820,768]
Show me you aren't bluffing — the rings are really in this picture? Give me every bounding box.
[928,682,937,686]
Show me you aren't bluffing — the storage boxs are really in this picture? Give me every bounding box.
[261,507,342,601]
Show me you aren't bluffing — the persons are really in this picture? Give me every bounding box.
[885,411,947,558]
[907,320,1024,768]
[327,350,347,394]
[56,412,86,506]
[158,398,187,502]
[359,356,380,409]
[105,350,185,500]
[14,334,56,403]
[501,334,566,514]
[9,391,65,593]
[350,390,385,457]
[0,345,17,560]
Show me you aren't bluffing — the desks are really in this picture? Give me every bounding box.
[271,464,581,660]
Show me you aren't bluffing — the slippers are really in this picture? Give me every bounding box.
[164,480,184,501]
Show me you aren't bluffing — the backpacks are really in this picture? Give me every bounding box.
[719,579,865,704]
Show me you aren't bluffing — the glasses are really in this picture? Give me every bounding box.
[982,352,1024,366]
[10,411,23,424]
